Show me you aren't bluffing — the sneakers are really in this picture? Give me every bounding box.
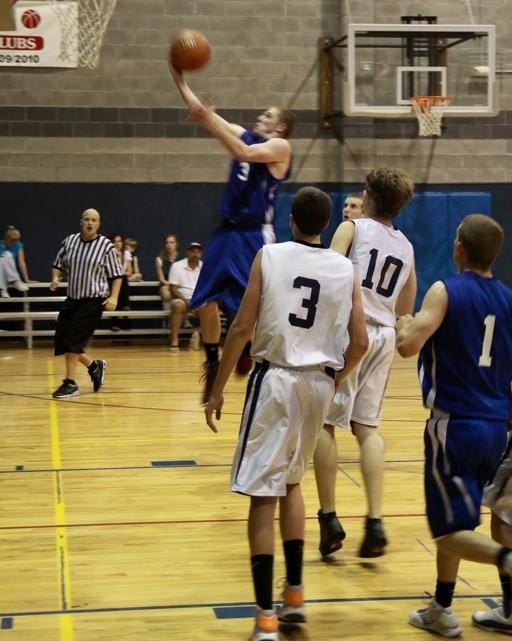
[276,578,307,622]
[53,379,80,398]
[14,281,29,291]
[358,516,388,558]
[183,319,194,329]
[236,340,253,376]
[499,551,511,617]
[408,590,463,638]
[87,360,107,393]
[1,290,10,298]
[170,343,180,351]
[317,509,346,556]
[248,605,279,641]
[471,599,512,635]
[198,362,219,407]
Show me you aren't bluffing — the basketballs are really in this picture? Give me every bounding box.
[172,30,211,70]
[21,9,41,28]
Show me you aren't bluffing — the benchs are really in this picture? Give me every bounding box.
[0,279,229,348]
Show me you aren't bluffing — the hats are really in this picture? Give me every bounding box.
[187,242,204,250]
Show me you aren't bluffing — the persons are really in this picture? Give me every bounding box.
[313,169,417,559]
[110,233,132,332]
[166,242,204,354]
[341,193,365,222]
[49,207,123,399]
[393,214,512,638]
[471,424,512,637]
[155,233,193,330]
[123,238,143,282]
[167,50,297,409]
[0,225,41,298]
[203,187,368,641]
